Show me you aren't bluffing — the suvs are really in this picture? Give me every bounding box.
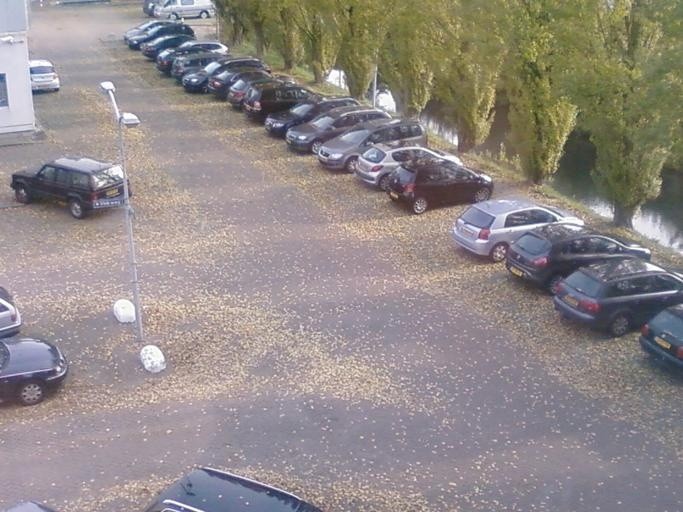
[503,223,653,294]
[546,256,683,338]
[4,150,134,221]
[138,461,324,512]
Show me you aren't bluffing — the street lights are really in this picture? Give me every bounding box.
[99,78,147,341]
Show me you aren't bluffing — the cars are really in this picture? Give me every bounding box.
[1,498,54,512]
[266,91,495,218]
[0,334,70,411]
[635,300,683,374]
[119,0,320,119]
[449,196,587,263]
[0,283,24,338]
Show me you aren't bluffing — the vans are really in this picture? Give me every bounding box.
[28,56,61,94]
